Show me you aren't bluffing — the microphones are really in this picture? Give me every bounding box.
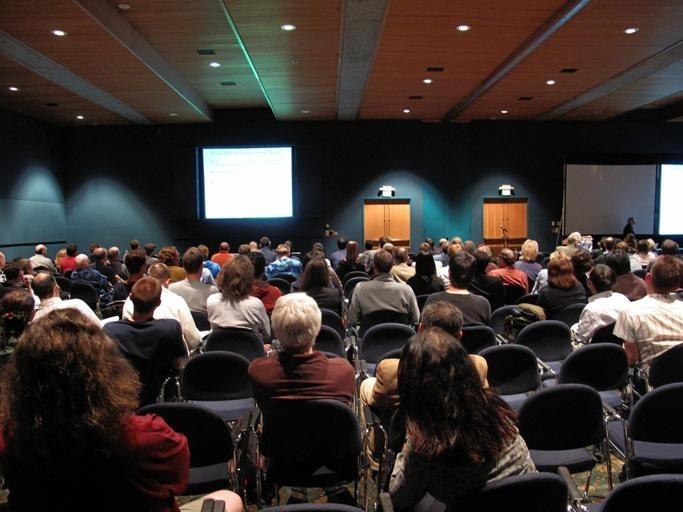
[501,224,509,234]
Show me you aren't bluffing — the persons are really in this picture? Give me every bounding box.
[0,217,683,512]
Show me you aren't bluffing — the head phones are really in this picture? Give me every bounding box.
[259,238,272,248]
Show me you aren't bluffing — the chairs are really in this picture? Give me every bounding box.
[0,252,683,511]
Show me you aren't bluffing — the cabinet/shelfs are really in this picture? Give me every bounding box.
[364,197,411,249]
[483,197,529,255]
